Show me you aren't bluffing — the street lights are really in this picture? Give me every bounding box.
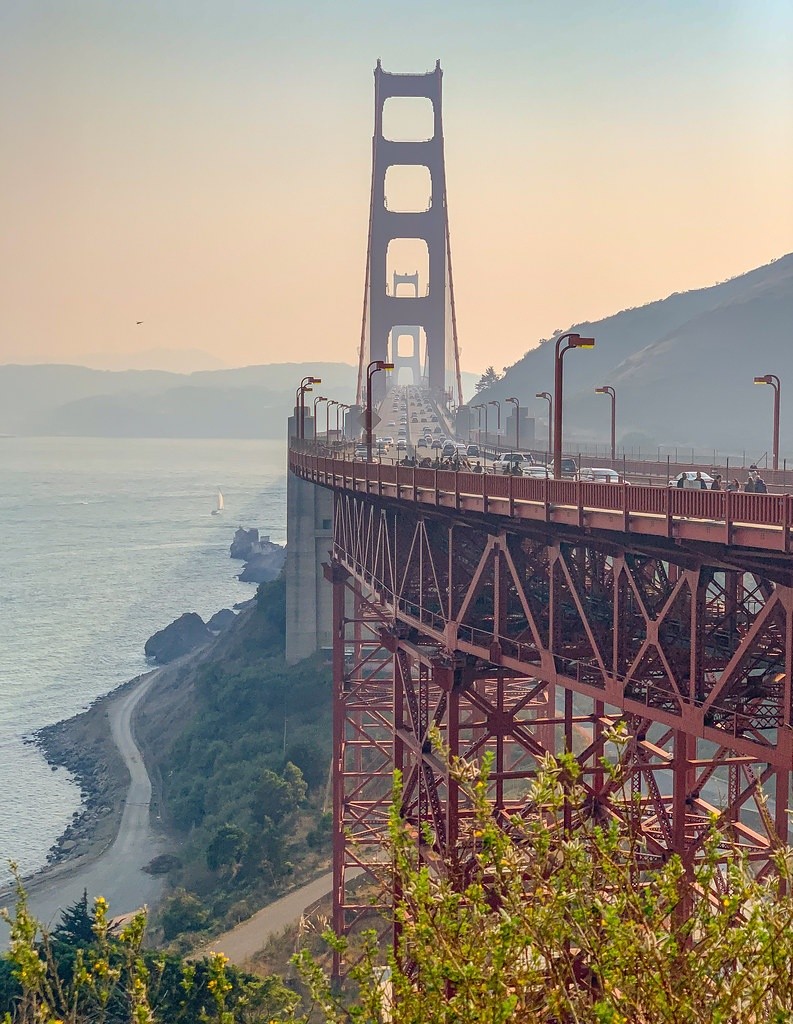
[365,360,394,463]
[314,396,327,448]
[554,334,596,482]
[296,387,313,450]
[335,403,347,443]
[341,405,351,441]
[476,404,488,445]
[594,385,615,468]
[488,401,501,446]
[535,392,551,453]
[754,374,779,471]
[300,376,321,453]
[505,398,519,452]
[325,400,338,447]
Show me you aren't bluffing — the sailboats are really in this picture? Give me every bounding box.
[210,489,224,515]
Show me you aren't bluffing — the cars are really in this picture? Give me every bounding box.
[353,384,483,463]
[572,468,630,487]
[522,467,554,480]
[492,454,529,470]
[669,472,714,490]
[547,458,576,476]
